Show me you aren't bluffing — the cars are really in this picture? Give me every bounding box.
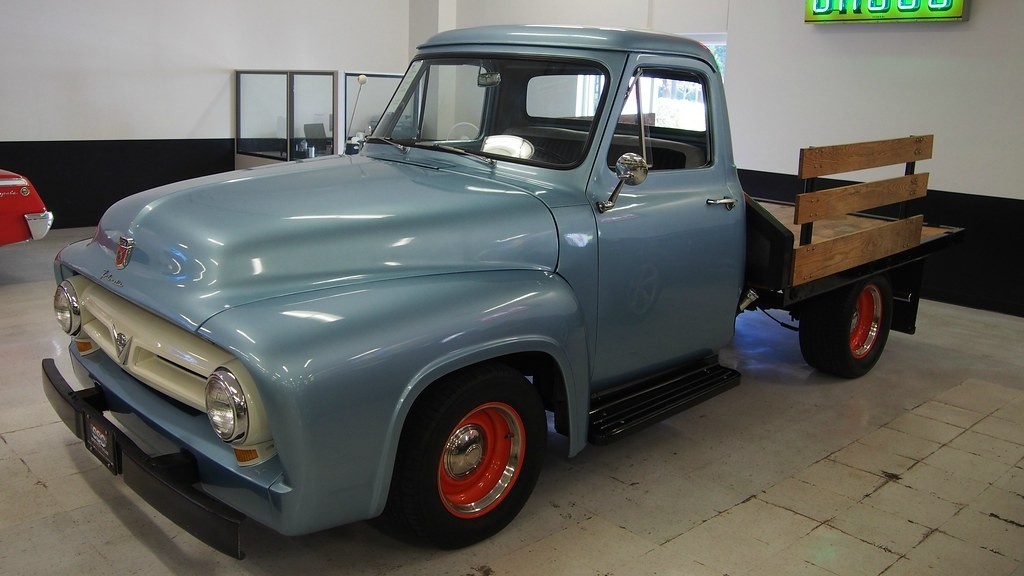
[0,169,53,249]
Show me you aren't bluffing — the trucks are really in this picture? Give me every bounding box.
[37,20,968,565]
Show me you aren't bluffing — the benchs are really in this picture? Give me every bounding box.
[500,126,707,169]
